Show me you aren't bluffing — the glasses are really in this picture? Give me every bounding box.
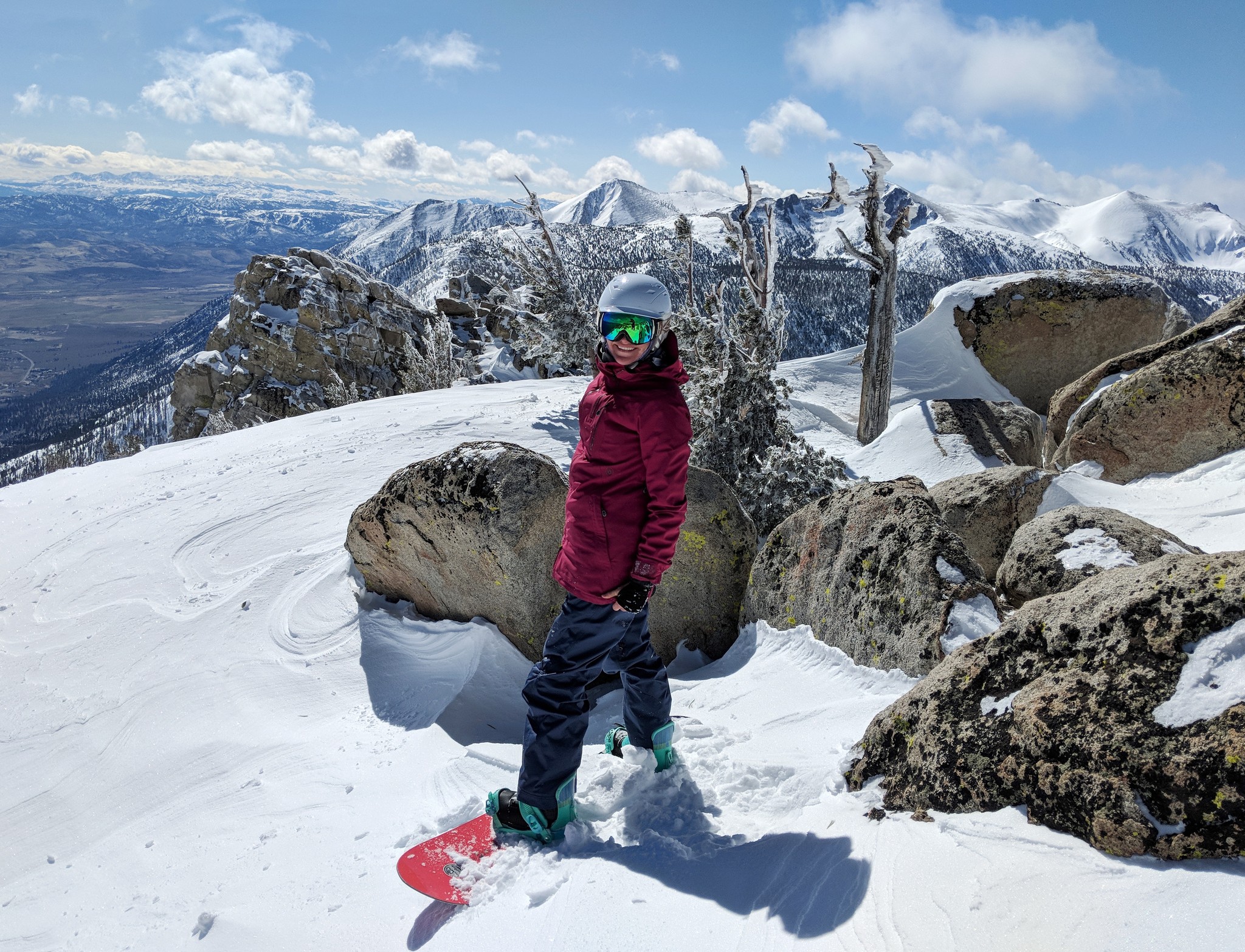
[599,313,663,344]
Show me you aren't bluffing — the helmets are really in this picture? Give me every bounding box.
[596,273,672,338]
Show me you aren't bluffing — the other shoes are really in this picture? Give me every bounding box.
[605,721,674,773]
[493,772,576,843]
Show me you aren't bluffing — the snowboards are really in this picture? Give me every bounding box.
[396,714,703,905]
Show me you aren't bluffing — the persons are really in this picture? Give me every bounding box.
[485,273,693,848]
